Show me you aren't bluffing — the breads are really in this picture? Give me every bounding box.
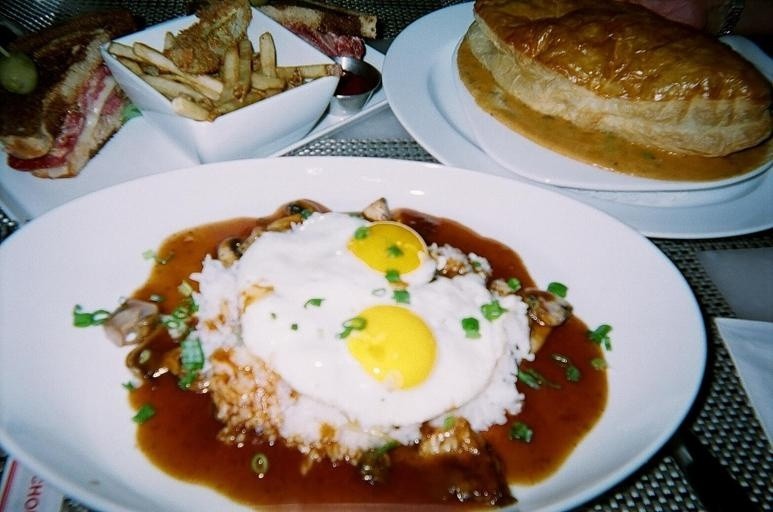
[466,0,773,157]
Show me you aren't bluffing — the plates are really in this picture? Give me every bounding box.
[381,0,773,240]
[2,38,399,226]
[0,154,710,510]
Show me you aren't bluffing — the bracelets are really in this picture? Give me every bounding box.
[714,0,744,38]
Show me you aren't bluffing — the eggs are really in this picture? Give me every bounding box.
[220,212,436,293]
[240,284,494,429]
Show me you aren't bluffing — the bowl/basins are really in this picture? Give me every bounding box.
[322,55,383,120]
[101,4,341,161]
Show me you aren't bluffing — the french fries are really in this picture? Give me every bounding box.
[111,33,343,121]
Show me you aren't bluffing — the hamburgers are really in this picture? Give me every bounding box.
[0,28,126,178]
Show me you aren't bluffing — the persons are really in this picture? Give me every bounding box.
[635,0,772,41]
